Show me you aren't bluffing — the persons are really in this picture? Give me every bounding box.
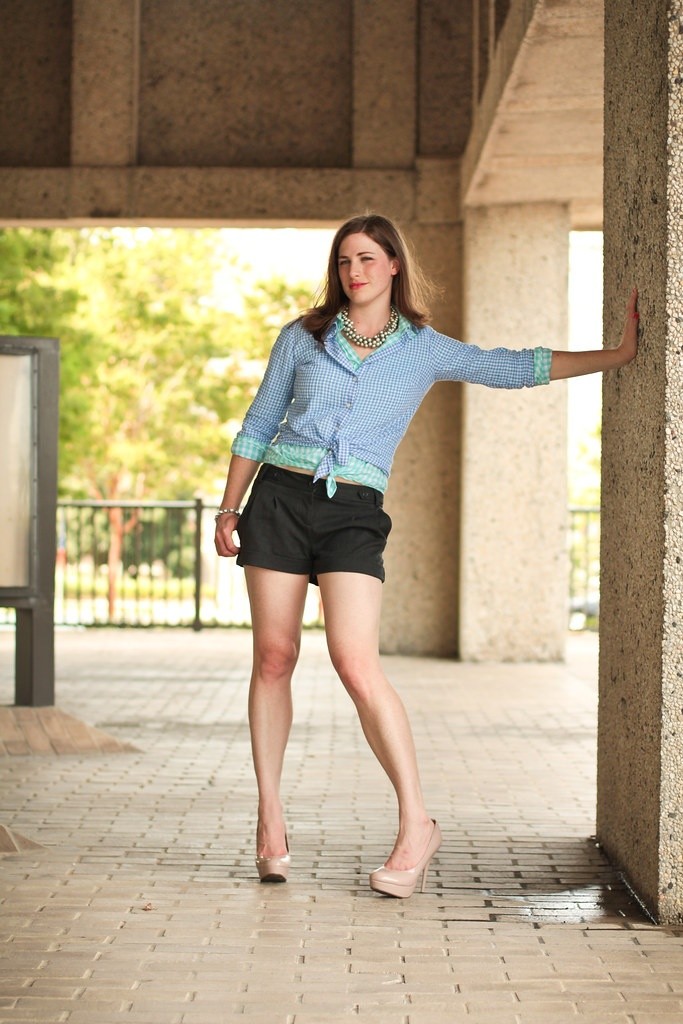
[211,213,640,899]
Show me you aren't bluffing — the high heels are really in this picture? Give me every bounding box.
[255,813,291,883]
[368,818,442,901]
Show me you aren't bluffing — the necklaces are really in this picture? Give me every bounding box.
[337,302,399,349]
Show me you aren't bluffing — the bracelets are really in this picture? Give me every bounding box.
[214,509,242,520]
